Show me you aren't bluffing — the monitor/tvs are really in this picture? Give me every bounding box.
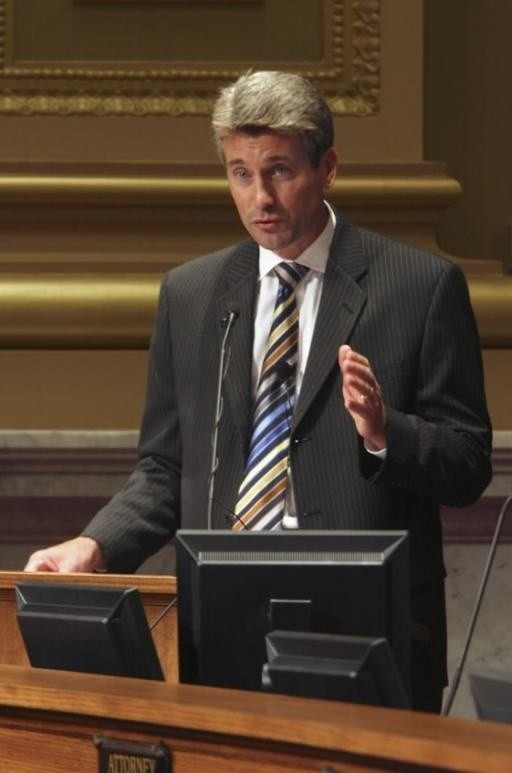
[176,529,412,710]
[13,581,166,684]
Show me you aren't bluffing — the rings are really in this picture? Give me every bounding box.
[360,386,375,402]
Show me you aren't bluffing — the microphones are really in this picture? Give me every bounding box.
[205,305,241,526]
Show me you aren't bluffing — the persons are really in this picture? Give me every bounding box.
[22,64,495,714]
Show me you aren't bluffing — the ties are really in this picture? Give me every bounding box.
[232,261,309,530]
